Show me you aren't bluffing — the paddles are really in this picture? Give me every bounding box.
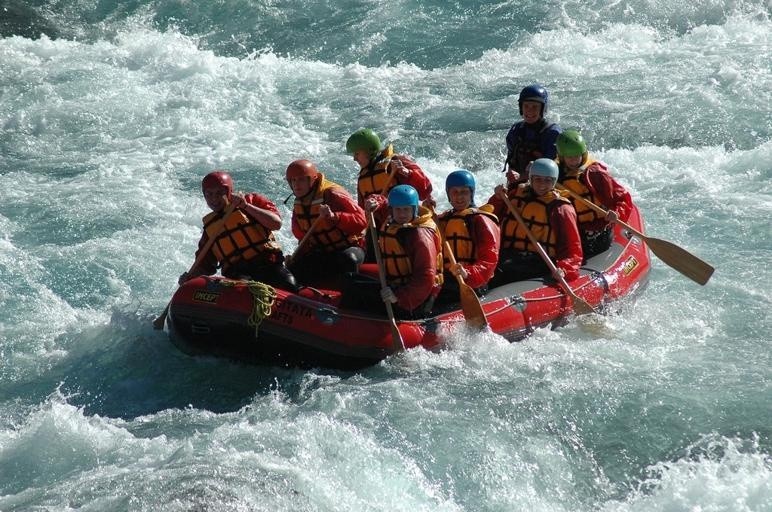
[423,193,487,333]
[495,184,607,334]
[526,161,715,287]
[363,204,407,353]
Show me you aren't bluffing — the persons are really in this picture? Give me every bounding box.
[364,183,445,322]
[501,83,563,190]
[282,158,368,287]
[176,170,300,294]
[553,128,627,264]
[484,156,584,291]
[338,127,432,264]
[420,169,503,318]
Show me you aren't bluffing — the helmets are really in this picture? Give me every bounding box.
[345,127,382,161]
[528,157,560,190]
[285,159,319,192]
[201,171,234,200]
[387,184,420,219]
[517,84,548,118]
[556,130,587,163]
[445,169,476,201]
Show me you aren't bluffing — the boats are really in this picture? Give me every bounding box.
[160,158,654,365]
